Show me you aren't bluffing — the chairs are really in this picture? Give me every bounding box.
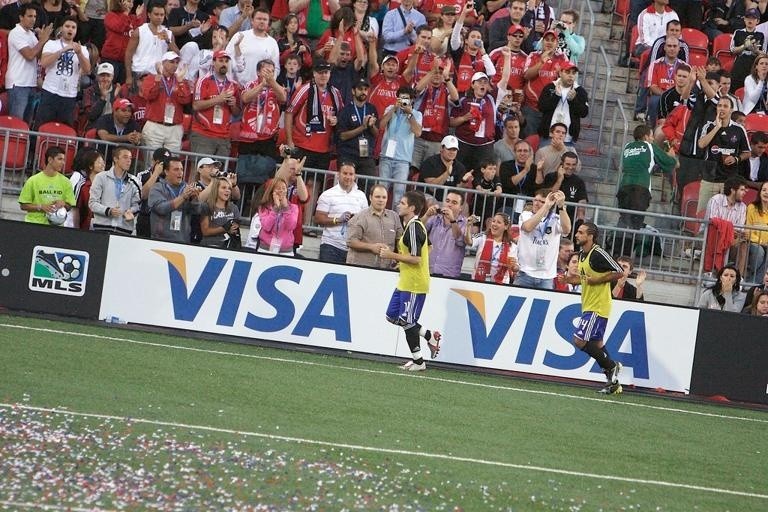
[742,189,758,206]
[734,87,744,102]
[0,92,9,116]
[743,114,768,154]
[33,122,78,176]
[85,129,97,149]
[609,0,630,40]
[626,25,643,93]
[0,116,29,168]
[681,28,709,69]
[633,48,652,121]
[679,181,702,235]
[178,115,194,161]
[324,159,337,189]
[713,33,736,72]
[523,134,539,154]
[688,210,706,275]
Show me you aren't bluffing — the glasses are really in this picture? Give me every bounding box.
[512,33,524,38]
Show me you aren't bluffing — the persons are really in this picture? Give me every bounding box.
[557,238,575,276]
[136,147,171,237]
[677,65,720,188]
[416,135,475,220]
[314,162,369,264]
[18,146,76,226]
[742,54,768,115]
[743,291,768,317]
[263,143,311,259]
[199,176,240,248]
[557,221,625,395]
[633,0,681,55]
[610,256,646,302]
[702,1,745,40]
[79,149,106,231]
[699,174,747,278]
[652,64,705,173]
[743,128,768,182]
[1,0,590,210]
[635,19,689,113]
[346,184,404,270]
[730,8,764,94]
[730,111,746,128]
[88,145,142,236]
[745,0,768,23]
[553,252,582,293]
[686,97,751,259]
[147,157,200,244]
[616,125,681,230]
[705,69,743,113]
[696,57,721,91]
[419,190,473,278]
[380,190,442,372]
[473,158,502,224]
[189,158,241,244]
[645,35,693,129]
[661,104,693,153]
[498,140,548,224]
[64,147,97,229]
[696,266,747,313]
[746,179,768,285]
[535,122,582,178]
[513,188,571,290]
[741,267,768,312]
[543,151,588,239]
[257,177,298,257]
[464,212,521,284]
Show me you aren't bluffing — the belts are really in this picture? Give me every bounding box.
[155,120,176,127]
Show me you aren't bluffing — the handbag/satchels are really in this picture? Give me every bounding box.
[397,6,414,46]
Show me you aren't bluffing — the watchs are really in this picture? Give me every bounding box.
[232,185,236,188]
[461,178,468,183]
[295,172,303,176]
[450,220,456,223]
[557,205,566,211]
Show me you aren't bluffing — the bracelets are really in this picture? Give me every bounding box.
[333,217,337,225]
[181,194,187,201]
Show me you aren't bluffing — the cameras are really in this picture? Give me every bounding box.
[285,148,291,156]
[437,208,442,214]
[400,98,410,107]
[161,160,165,178]
[555,21,565,30]
[474,216,481,222]
[218,171,231,179]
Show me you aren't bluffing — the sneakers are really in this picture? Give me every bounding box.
[596,382,622,395]
[602,361,623,387]
[427,330,442,359]
[398,361,427,371]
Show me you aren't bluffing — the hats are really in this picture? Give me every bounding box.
[440,135,460,152]
[152,147,170,160]
[213,49,232,62]
[112,98,135,110]
[441,5,456,15]
[197,157,222,171]
[560,61,579,72]
[352,78,369,87]
[430,59,444,69]
[313,58,331,73]
[543,30,557,38]
[509,24,524,34]
[470,71,490,83]
[97,62,114,76]
[161,51,182,62]
[745,8,760,19]
[382,54,399,67]
